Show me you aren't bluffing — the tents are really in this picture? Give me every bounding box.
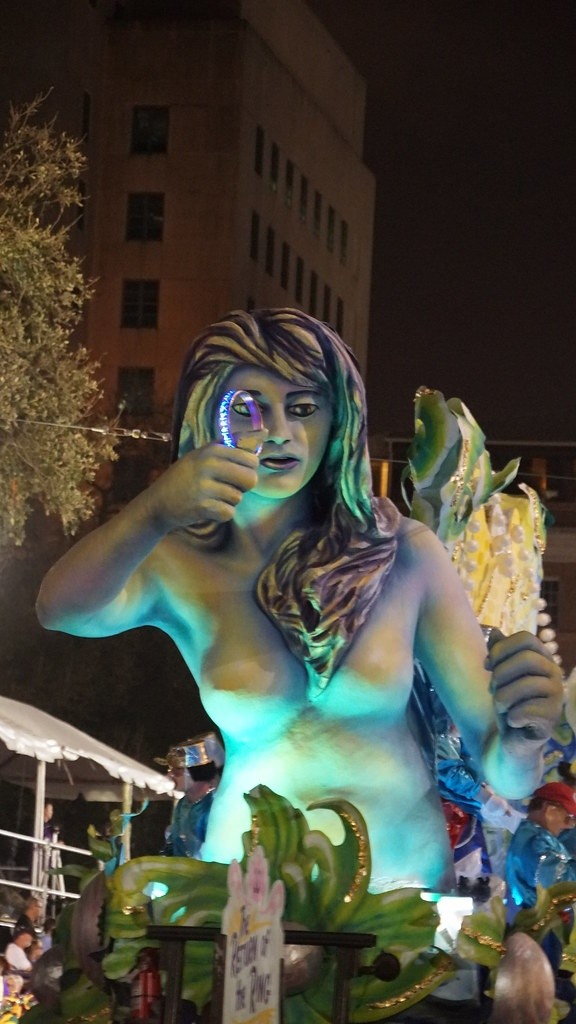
[0,695,185,933]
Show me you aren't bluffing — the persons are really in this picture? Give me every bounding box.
[0,896,56,999]
[165,728,226,858]
[35,309,564,896]
[434,717,576,974]
[42,802,64,846]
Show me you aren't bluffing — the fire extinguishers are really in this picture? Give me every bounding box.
[128,947,160,1024]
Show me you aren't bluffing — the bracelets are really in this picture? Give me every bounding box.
[219,389,263,458]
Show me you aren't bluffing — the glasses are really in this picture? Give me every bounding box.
[168,766,189,777]
[564,816,576,824]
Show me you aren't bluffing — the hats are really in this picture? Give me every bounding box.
[154,731,222,768]
[533,782,576,814]
[13,923,40,939]
